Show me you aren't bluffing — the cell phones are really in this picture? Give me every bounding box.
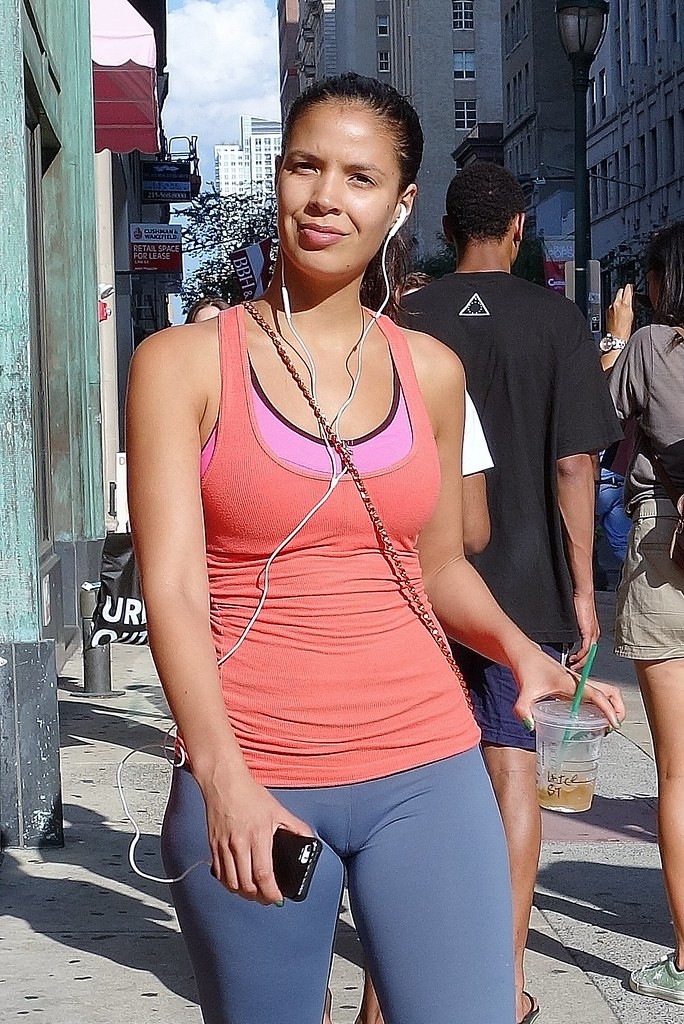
[207,826,322,903]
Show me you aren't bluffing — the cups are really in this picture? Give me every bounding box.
[530,700,610,814]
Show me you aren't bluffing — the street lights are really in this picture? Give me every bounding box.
[554,0,610,313]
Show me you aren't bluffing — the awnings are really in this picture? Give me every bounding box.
[90,0,161,155]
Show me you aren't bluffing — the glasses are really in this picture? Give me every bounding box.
[634,268,652,309]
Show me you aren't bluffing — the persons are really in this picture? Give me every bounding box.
[600,222,684,1004]
[355,162,626,1023]
[186,296,231,326]
[124,71,626,1023]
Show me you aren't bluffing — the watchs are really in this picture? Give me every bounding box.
[598,334,626,352]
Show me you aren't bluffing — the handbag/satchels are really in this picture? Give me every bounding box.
[82,532,150,652]
[668,493,684,570]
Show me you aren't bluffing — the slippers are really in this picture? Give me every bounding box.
[521,991,540,1024]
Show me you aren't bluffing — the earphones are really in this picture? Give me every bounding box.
[388,203,407,236]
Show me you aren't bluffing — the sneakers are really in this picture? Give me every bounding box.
[629,952,684,1005]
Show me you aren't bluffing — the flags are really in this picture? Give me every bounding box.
[230,238,272,300]
